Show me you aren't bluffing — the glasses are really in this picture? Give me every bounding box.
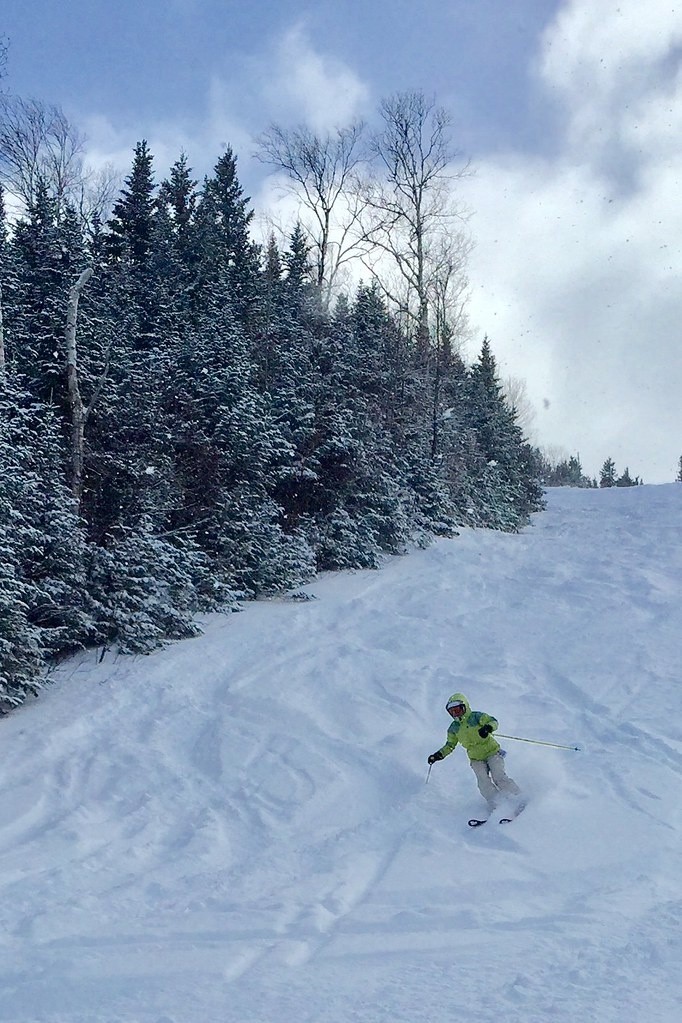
[449,707,461,714]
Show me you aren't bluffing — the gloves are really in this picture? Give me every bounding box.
[478,724,492,738]
[428,751,444,765]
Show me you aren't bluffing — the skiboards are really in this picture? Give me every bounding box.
[468,803,527,827]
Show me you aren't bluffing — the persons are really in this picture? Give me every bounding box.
[428,693,520,805]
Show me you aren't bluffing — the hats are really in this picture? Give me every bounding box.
[446,701,462,710]
